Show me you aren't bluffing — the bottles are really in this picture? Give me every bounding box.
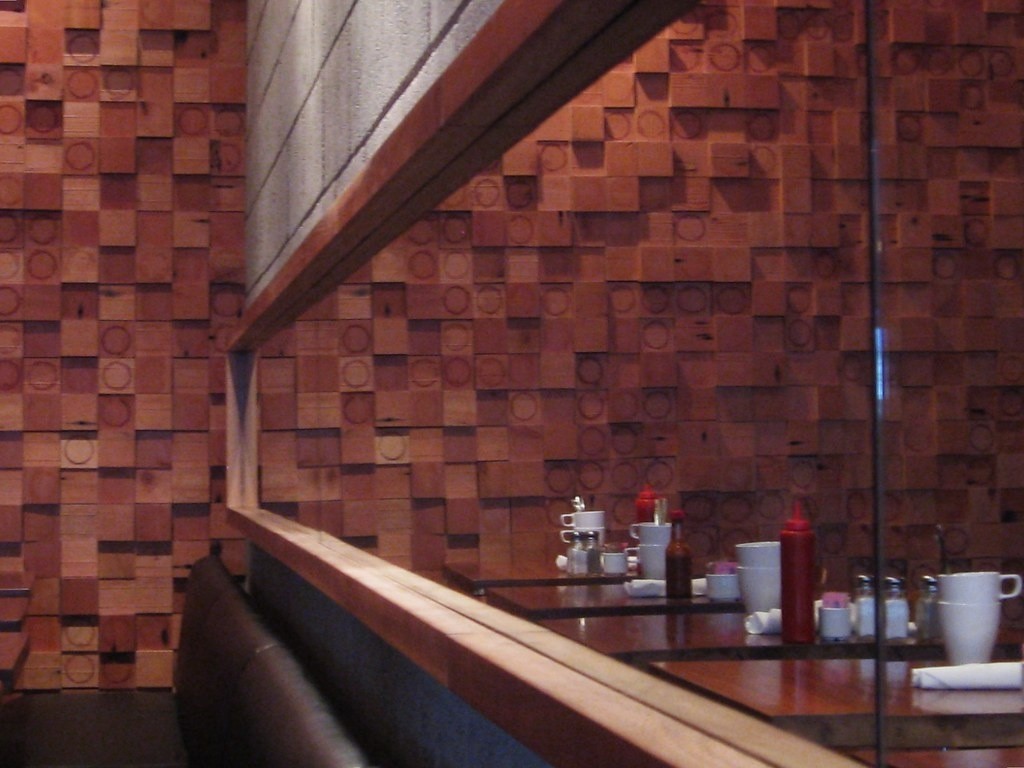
[857,575,937,643]
[636,485,657,522]
[585,532,601,571]
[782,497,814,642]
[666,518,691,599]
[567,532,585,574]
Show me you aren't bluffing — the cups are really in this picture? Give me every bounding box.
[561,511,607,547]
[602,553,627,576]
[706,542,782,615]
[818,606,854,638]
[628,521,671,576]
[938,572,1023,663]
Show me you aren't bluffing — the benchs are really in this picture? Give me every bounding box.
[0,555,367,766]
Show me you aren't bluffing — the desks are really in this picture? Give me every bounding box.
[642,658,1024,752]
[443,553,642,587]
[542,614,1024,660]
[485,579,776,614]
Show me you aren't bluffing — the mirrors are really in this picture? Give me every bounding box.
[225,0,1022,767]
